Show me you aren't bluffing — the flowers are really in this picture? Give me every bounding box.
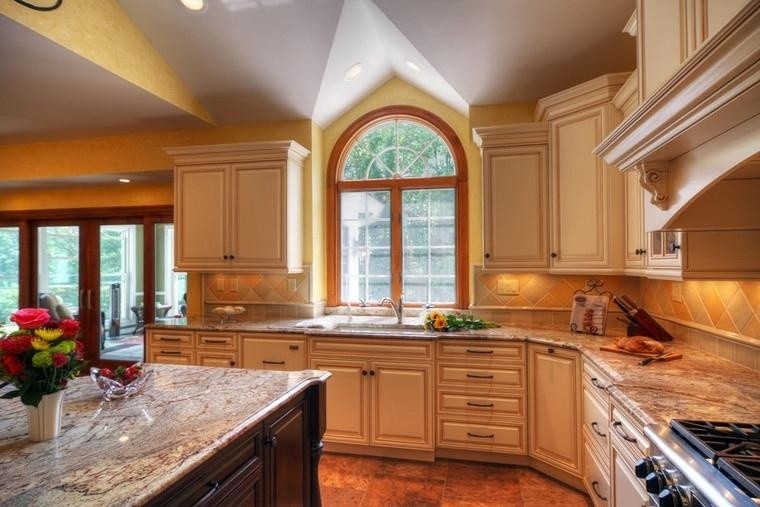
[0,308,91,409]
[423,312,500,331]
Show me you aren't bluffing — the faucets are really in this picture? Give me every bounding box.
[377,297,401,324]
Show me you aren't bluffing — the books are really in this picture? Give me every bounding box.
[569,295,609,336]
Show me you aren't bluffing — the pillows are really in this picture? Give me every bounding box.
[58,306,74,320]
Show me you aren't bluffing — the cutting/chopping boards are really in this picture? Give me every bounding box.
[599,344,683,362]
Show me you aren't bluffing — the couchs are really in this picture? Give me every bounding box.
[40,293,105,348]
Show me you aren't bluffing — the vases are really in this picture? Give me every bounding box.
[23,391,67,444]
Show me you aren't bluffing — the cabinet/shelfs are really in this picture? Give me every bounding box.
[432,344,526,458]
[637,1,697,106]
[698,0,760,47]
[134,420,263,507]
[311,337,433,445]
[610,394,652,506]
[529,345,580,473]
[581,360,612,507]
[547,115,612,270]
[264,397,314,506]
[647,232,760,279]
[484,146,547,269]
[175,165,284,271]
[239,334,310,370]
[144,329,239,368]
[621,94,647,272]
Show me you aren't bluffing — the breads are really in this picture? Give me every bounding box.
[214,306,245,316]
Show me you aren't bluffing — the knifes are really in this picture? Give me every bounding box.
[611,294,638,316]
[639,350,674,366]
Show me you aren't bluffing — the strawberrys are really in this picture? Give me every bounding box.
[93,364,142,387]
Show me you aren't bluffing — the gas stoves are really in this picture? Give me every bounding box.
[633,417,760,506]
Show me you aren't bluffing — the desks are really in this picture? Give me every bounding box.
[132,304,173,336]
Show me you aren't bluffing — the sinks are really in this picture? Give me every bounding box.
[332,322,426,335]
